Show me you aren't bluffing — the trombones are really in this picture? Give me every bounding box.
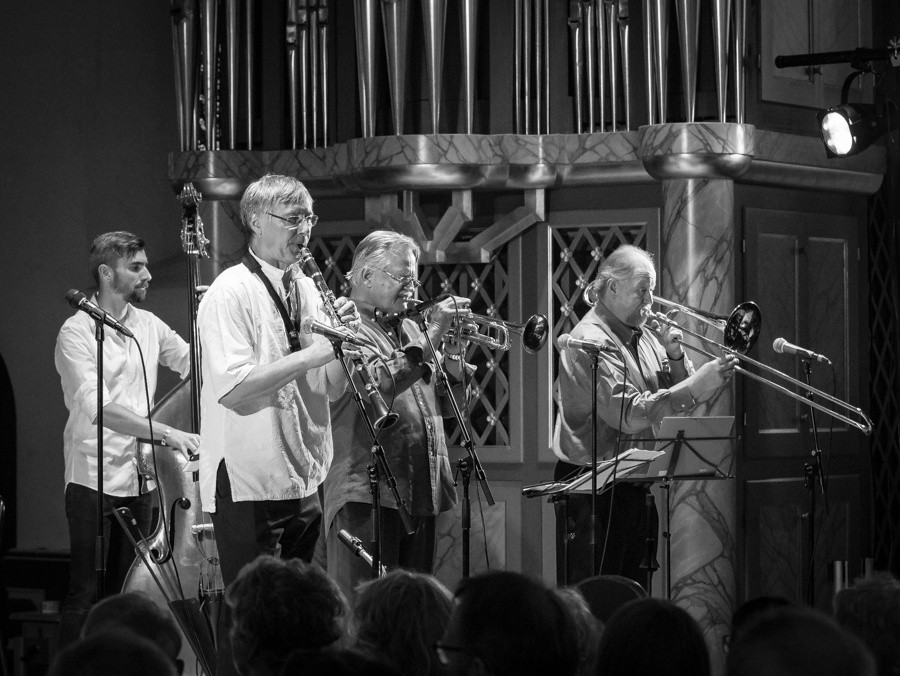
[581,281,874,435]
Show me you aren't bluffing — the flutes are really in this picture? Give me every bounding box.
[298,242,400,433]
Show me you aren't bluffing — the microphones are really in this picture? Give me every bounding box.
[303,316,365,347]
[65,287,135,337]
[773,337,831,364]
[557,332,618,354]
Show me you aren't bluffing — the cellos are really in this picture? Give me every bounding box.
[115,183,225,676]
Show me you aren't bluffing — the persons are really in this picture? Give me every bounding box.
[197,173,365,673]
[2,552,899,676]
[323,231,475,613]
[548,241,741,600]
[54,230,200,636]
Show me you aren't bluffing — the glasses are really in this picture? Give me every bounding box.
[373,265,421,288]
[264,211,318,230]
[435,640,478,668]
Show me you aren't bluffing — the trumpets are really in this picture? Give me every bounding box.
[407,295,552,354]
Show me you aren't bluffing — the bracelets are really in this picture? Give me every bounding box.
[692,398,696,404]
[433,320,447,334]
[160,426,172,445]
[444,350,465,361]
[666,351,684,362]
